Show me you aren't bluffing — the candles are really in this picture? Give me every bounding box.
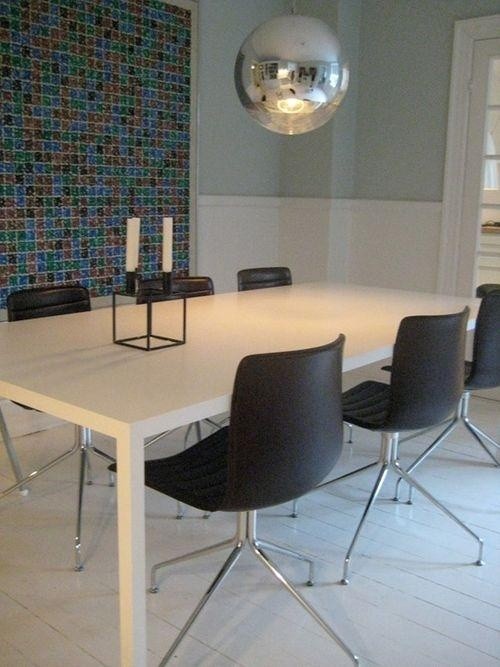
[124,215,142,270]
[161,215,174,271]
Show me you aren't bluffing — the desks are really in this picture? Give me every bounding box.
[0,278,481,667]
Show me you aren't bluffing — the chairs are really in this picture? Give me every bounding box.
[391,283,500,504]
[135,275,215,519]
[238,266,355,443]
[291,304,484,585]
[106,334,358,666]
[2,288,183,572]
